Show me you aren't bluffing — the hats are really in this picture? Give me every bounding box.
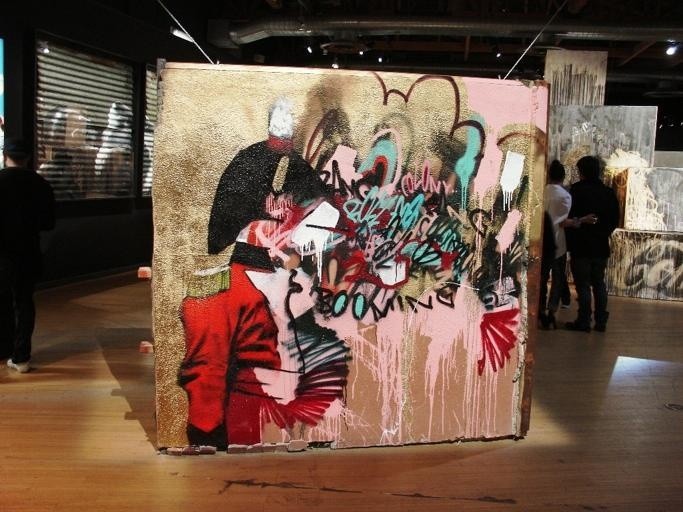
[4,136,29,152]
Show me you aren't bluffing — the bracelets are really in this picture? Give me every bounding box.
[574,216,581,229]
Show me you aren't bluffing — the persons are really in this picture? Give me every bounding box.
[560,270,572,309]
[205,98,330,271]
[563,154,621,333]
[0,136,59,373]
[538,160,600,329]
[36,100,153,198]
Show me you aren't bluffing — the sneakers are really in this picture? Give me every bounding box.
[7,359,31,373]
